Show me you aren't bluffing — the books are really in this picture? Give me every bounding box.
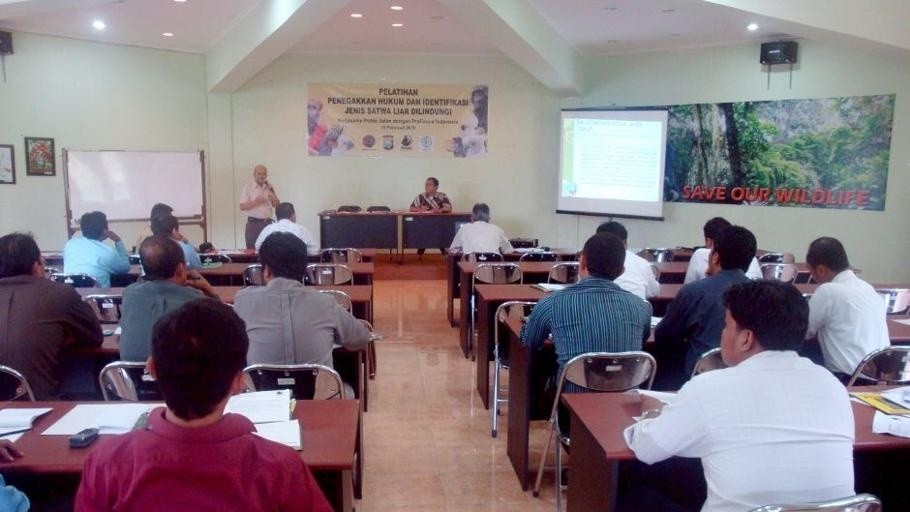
[0,406,54,443]
[850,384,910,441]
[529,281,569,293]
[222,388,303,456]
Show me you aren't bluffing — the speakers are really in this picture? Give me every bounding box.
[757,42,799,67]
[0,31,15,58]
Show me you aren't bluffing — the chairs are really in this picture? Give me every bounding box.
[44,267,59,279]
[129,254,143,266]
[198,254,232,264]
[802,293,814,302]
[807,274,818,284]
[472,263,523,361]
[460,251,504,262]
[491,300,538,437]
[241,363,346,400]
[760,263,799,285]
[649,262,661,283]
[635,247,674,263]
[574,248,582,262]
[548,262,582,284]
[84,294,123,324]
[337,205,362,212]
[366,206,392,255]
[757,252,784,264]
[533,351,656,512]
[519,250,561,262]
[49,273,102,288]
[0,366,36,403]
[690,347,728,379]
[846,345,910,387]
[243,265,267,286]
[747,491,882,512]
[320,247,362,263]
[318,290,353,315]
[302,264,354,286]
[99,361,163,402]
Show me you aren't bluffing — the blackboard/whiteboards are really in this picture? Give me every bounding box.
[66,148,203,224]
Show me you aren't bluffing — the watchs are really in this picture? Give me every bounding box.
[639,410,662,421]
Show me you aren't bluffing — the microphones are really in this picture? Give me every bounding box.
[265,181,275,194]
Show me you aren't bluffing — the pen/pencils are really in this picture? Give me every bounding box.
[189,276,201,280]
[1,427,34,438]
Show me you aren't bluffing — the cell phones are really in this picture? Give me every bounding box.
[66,427,101,448]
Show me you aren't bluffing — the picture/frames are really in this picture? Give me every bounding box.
[0,144,17,184]
[25,137,56,176]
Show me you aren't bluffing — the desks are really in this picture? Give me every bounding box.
[504,315,910,491]
[473,284,910,410]
[71,285,377,412]
[444,248,779,329]
[41,251,376,266]
[318,210,399,261]
[457,262,863,359]
[562,384,910,512]
[58,323,364,499]
[0,399,361,512]
[399,211,489,261]
[44,262,374,286]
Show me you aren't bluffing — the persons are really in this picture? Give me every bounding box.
[308,97,338,156]
[153,218,203,274]
[117,233,224,363]
[652,223,767,392]
[681,215,764,284]
[620,278,857,512]
[408,176,455,253]
[518,228,653,485]
[454,84,488,157]
[141,203,181,235]
[575,220,662,304]
[2,231,105,405]
[233,229,374,400]
[48,225,52,230]
[0,436,33,512]
[61,211,132,290]
[252,200,321,261]
[801,235,893,384]
[447,202,513,259]
[238,164,284,251]
[72,294,335,510]
